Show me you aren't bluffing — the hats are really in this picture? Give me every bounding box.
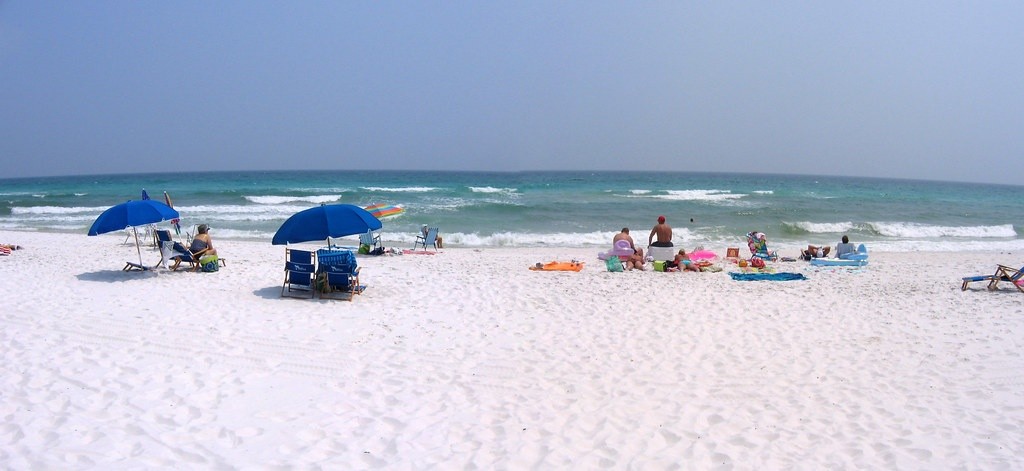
[657,216,665,222]
[198,224,210,231]
[817,248,823,258]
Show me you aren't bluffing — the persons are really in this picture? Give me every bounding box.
[614,228,646,271]
[842,235,856,252]
[674,245,704,273]
[164,224,222,267]
[423,226,444,248]
[647,215,674,248]
[798,244,831,261]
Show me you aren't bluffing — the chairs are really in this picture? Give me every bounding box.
[186,223,210,246]
[359,231,384,252]
[961,263,1024,291]
[153,229,195,269]
[162,241,226,272]
[280,248,317,299]
[317,249,367,302]
[413,227,439,251]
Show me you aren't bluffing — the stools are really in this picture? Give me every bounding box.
[649,247,674,261]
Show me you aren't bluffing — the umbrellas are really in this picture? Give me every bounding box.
[271,203,383,250]
[88,199,180,272]
[363,203,406,250]
[141,189,150,198]
[161,191,185,243]
